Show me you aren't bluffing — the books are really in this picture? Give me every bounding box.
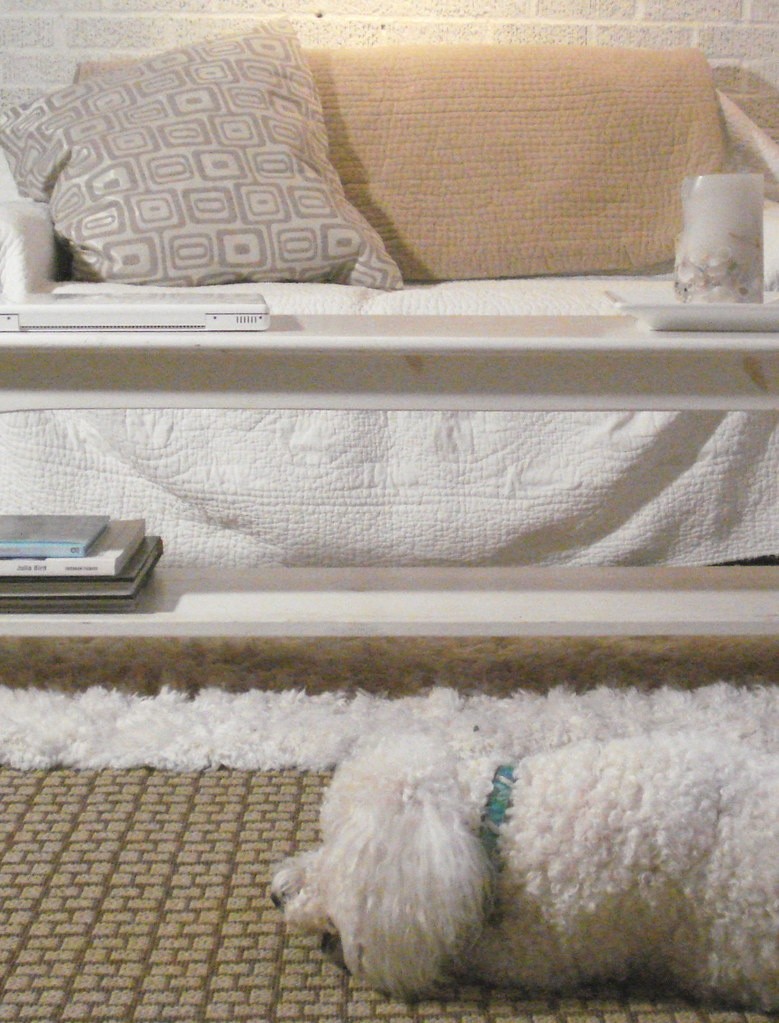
[0,514,164,613]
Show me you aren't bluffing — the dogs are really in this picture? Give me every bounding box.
[267,736,779,1015]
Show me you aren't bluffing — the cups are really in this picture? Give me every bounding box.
[673,174,763,306]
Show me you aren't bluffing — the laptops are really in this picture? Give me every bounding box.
[0,292,271,332]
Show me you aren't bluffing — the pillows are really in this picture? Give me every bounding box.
[0,20,404,290]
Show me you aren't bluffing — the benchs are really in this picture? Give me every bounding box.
[0,33,779,567]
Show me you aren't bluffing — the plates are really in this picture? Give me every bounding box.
[619,302,779,333]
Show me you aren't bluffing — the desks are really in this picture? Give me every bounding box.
[0,307,779,638]
